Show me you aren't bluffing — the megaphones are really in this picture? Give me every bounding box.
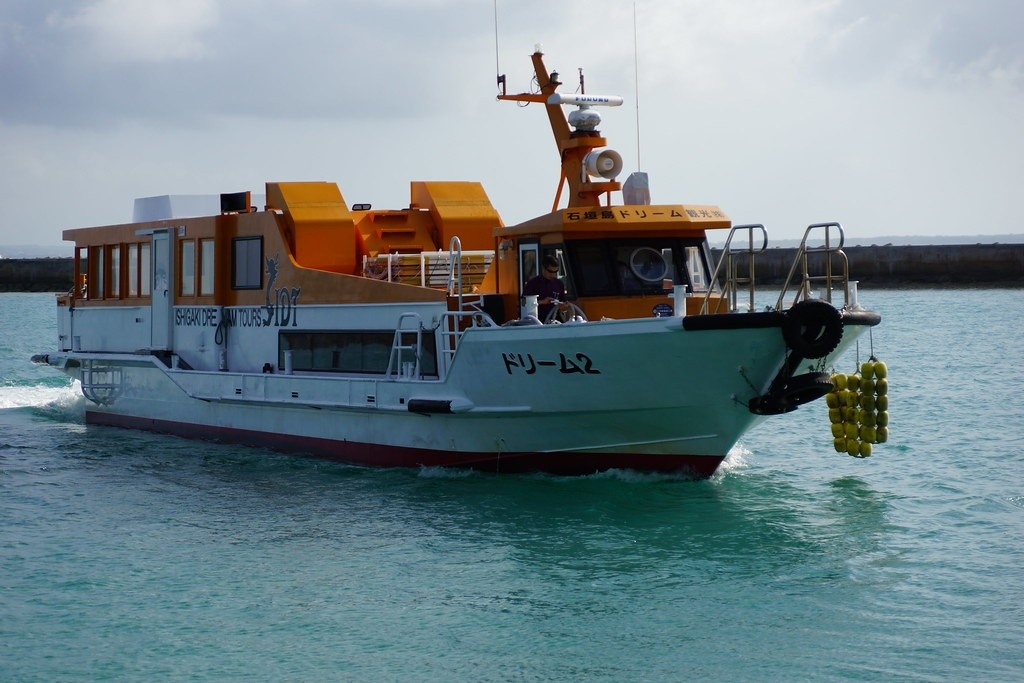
[587,148,623,178]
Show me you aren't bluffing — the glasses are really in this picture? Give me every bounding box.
[546,266,560,273]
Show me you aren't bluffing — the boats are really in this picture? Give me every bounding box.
[30,1,888,479]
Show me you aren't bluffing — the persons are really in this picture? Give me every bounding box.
[521,254,572,325]
